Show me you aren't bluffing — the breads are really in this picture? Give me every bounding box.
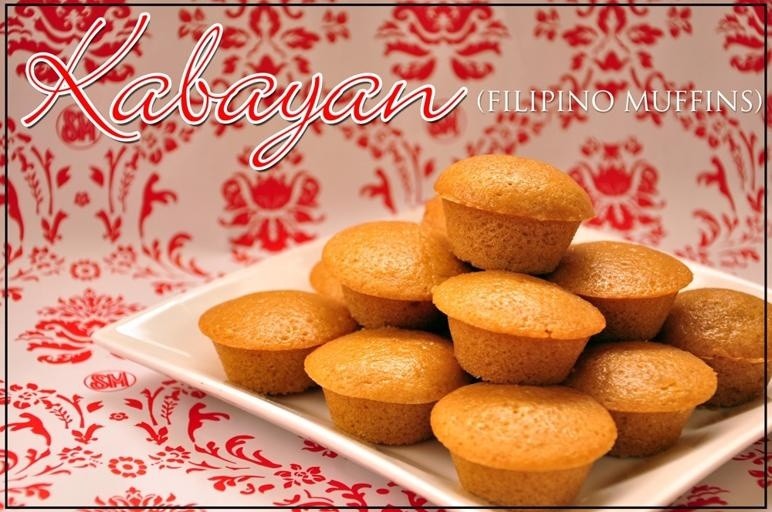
[198,152,772,512]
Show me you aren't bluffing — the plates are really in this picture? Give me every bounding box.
[91,211,772,512]
